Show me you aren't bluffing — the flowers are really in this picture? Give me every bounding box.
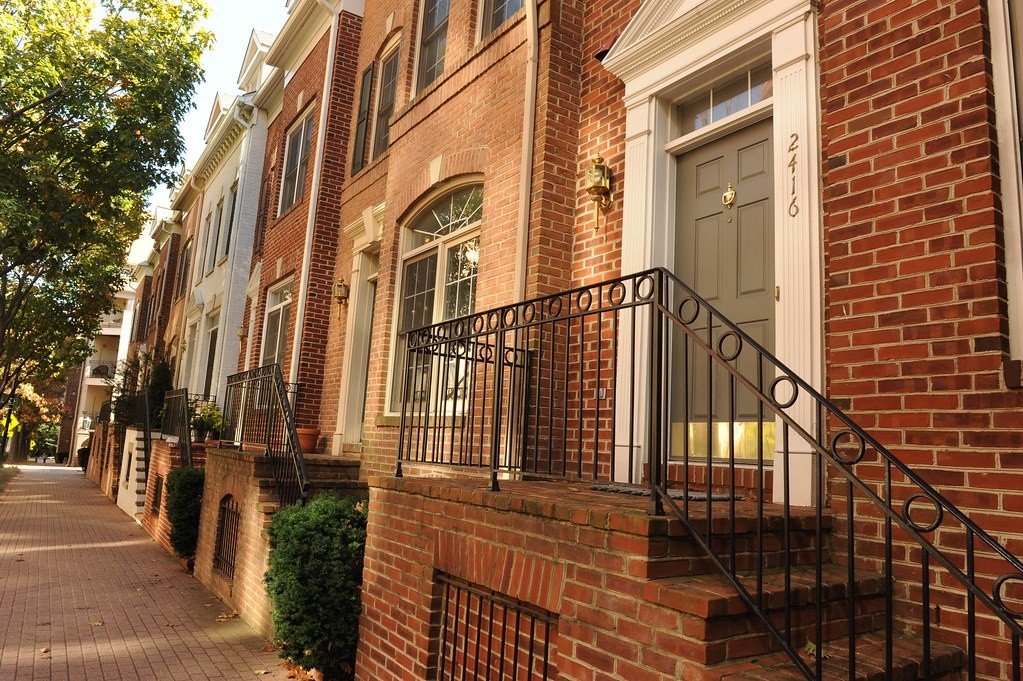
[192,402,223,432]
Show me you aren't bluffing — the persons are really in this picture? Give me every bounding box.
[34,448,48,463]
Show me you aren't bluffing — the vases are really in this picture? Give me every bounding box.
[193,429,208,443]
[296,428,321,453]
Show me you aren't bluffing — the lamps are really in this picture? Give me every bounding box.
[332,275,348,320]
[236,322,248,355]
[584,149,614,234]
